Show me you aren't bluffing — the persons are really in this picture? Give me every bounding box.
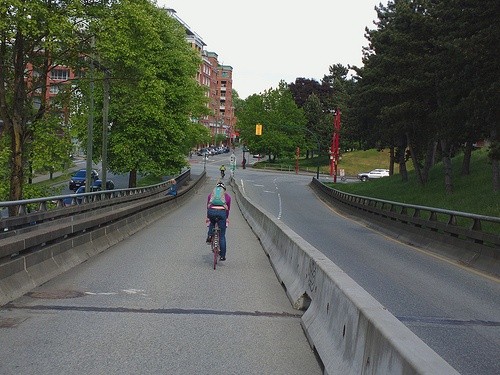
[219,164,226,177]
[258,154,261,162]
[241,157,246,169]
[206,181,231,261]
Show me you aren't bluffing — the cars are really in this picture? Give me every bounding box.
[74,185,105,203]
[90,179,115,190]
[62,196,83,207]
[357,168,390,182]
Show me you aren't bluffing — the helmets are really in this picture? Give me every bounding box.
[216,183,226,190]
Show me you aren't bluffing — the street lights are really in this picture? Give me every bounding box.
[302,127,320,180]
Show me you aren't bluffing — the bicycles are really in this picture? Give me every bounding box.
[219,171,226,180]
[205,217,230,271]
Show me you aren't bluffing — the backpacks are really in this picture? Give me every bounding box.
[210,187,228,210]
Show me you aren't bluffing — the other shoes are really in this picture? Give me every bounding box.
[207,236,211,242]
[220,256,226,261]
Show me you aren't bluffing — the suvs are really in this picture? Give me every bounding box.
[68,169,100,192]
